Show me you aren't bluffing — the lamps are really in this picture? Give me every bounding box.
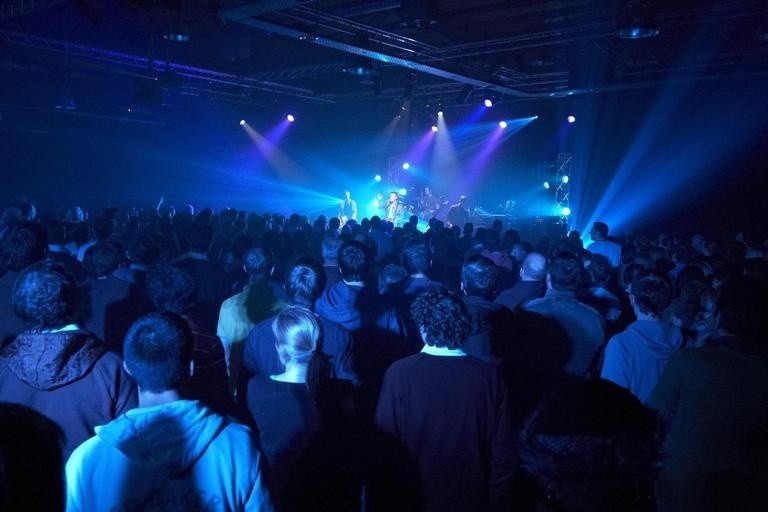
[611,0,661,39]
[363,67,503,109]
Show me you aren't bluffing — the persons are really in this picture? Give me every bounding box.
[1,187,766,511]
